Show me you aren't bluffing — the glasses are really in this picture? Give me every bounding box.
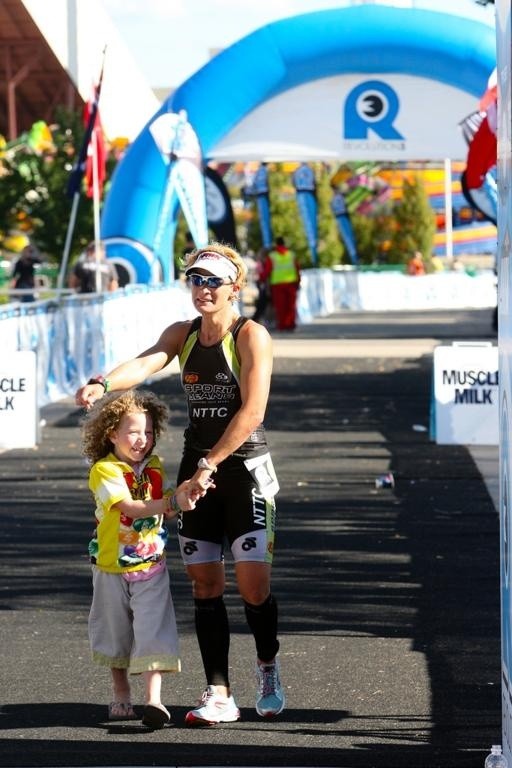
[189,274,231,288]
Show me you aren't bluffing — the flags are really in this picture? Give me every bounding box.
[81,93,107,202]
[65,84,103,203]
[455,61,497,225]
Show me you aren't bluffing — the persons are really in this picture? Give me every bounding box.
[12,243,46,302]
[232,236,300,331]
[330,185,358,265]
[253,161,273,250]
[80,386,217,725]
[77,241,287,726]
[293,161,317,269]
[68,240,119,292]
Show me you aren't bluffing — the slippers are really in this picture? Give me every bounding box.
[108,701,137,720]
[142,703,172,729]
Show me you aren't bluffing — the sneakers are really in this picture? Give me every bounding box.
[256,657,285,717]
[184,685,241,725]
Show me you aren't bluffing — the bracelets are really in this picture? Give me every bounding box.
[88,379,106,392]
[96,375,109,392]
[197,458,218,473]
[165,495,179,514]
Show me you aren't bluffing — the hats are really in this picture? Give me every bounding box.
[185,251,238,285]
